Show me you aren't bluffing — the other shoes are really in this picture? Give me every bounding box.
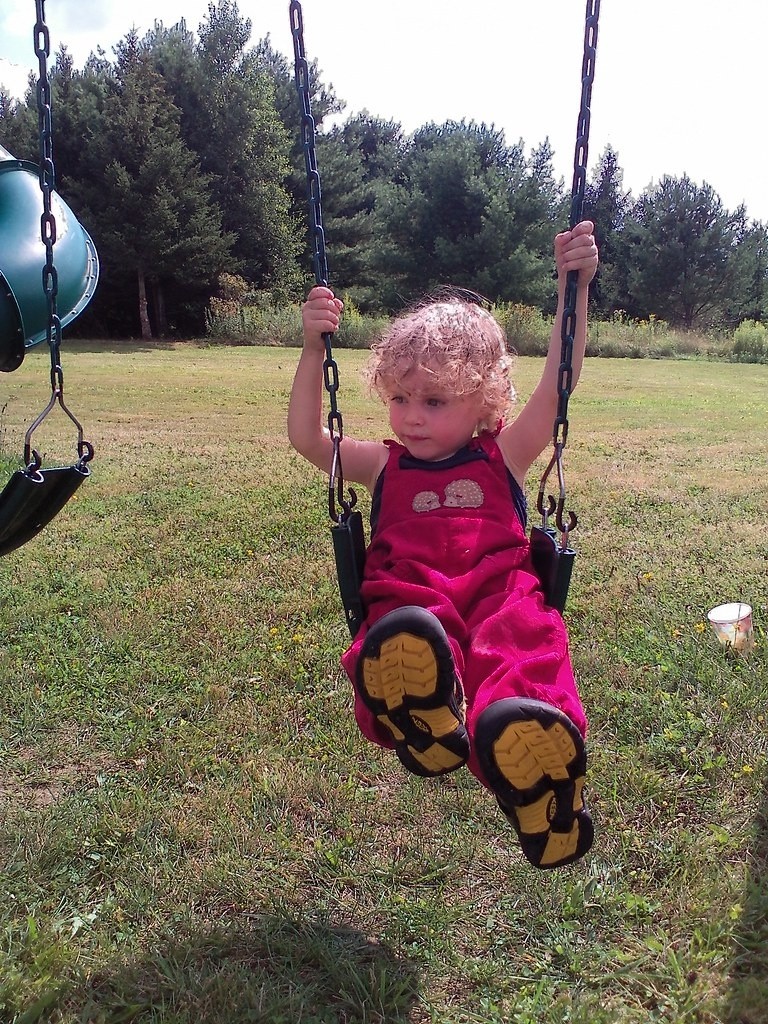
[355,607,470,777]
[474,699,594,870]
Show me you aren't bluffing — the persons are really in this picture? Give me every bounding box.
[287,220,598,869]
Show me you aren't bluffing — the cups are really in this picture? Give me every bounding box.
[707,603,755,657]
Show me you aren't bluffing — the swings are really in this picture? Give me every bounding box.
[0,0,97,562]
[290,0,606,643]
